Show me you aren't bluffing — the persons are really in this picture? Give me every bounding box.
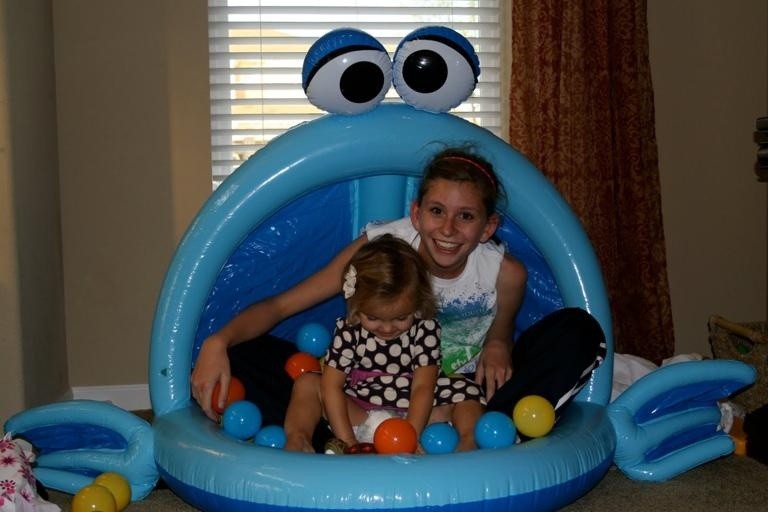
[187,140,606,440]
[275,234,481,454]
